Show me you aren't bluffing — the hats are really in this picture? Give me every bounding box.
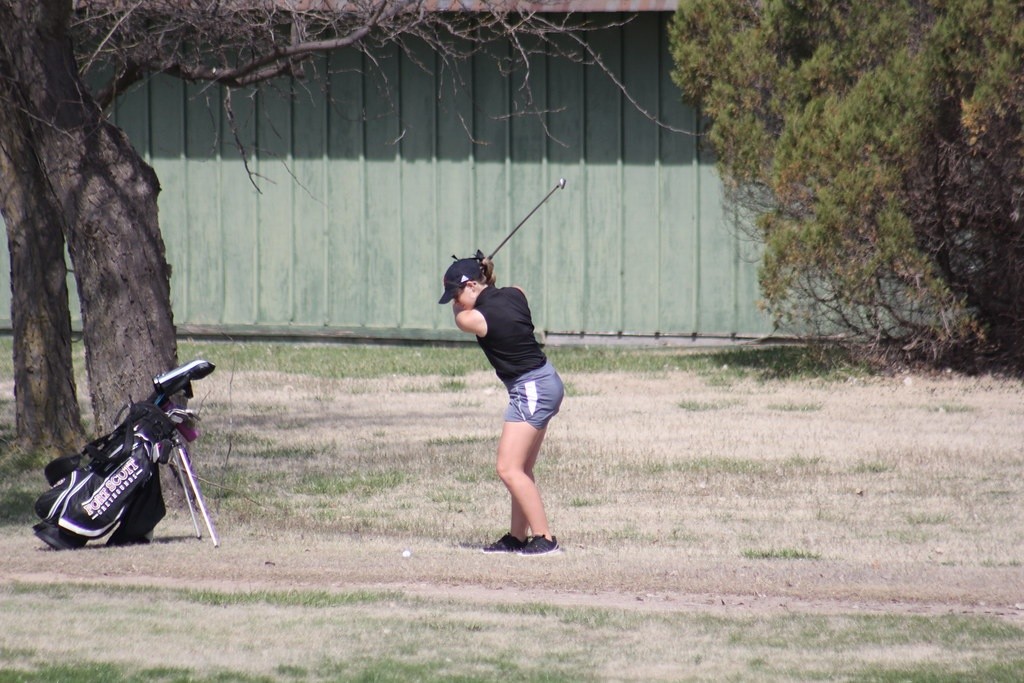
[440,258,480,303]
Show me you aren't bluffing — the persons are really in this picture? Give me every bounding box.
[438,256,566,554]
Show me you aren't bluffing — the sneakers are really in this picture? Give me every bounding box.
[516,536,559,556]
[481,533,528,555]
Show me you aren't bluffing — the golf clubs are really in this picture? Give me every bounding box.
[486,178,568,261]
[163,407,202,424]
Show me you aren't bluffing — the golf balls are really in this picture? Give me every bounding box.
[402,551,411,559]
